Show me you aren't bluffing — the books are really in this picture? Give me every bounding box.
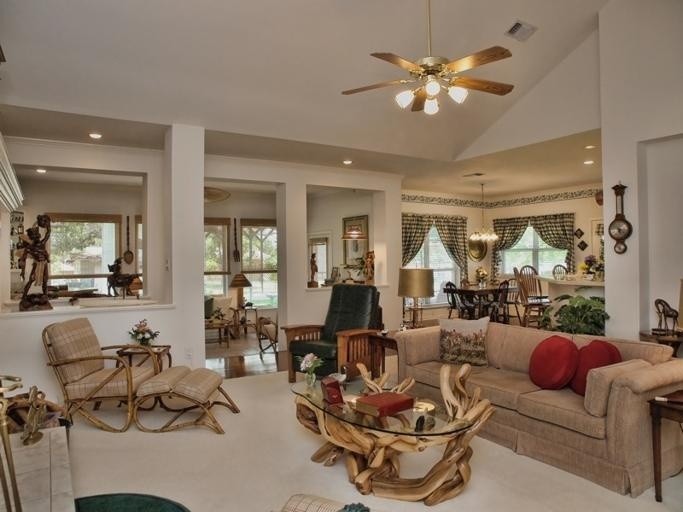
[353,391,414,419]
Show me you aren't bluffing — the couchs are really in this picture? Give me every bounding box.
[393,319,683,496]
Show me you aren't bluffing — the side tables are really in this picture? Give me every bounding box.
[647,388,683,503]
[115,344,172,407]
[368,329,400,384]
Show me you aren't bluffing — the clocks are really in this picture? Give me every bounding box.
[609,182,633,252]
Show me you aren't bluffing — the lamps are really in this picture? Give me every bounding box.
[395,76,470,115]
[228,273,253,288]
[469,183,500,243]
[397,268,435,329]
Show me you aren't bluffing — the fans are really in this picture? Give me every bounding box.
[341,0,514,112]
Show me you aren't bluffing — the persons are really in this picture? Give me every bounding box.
[112,257,122,275]
[311,253,316,282]
[20,215,51,300]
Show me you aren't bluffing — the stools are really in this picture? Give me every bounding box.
[133,366,240,434]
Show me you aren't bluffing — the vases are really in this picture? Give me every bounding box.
[582,271,594,281]
[139,337,150,346]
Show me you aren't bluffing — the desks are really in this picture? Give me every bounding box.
[0,426,77,512]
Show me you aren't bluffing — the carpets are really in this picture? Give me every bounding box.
[61,356,683,512]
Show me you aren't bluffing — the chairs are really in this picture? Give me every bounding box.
[42,317,161,432]
[205,295,279,353]
[280,284,386,383]
[443,264,568,329]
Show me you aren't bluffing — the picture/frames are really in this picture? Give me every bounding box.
[342,216,369,265]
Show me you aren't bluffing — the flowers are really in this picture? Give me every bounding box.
[127,319,160,343]
[578,255,597,274]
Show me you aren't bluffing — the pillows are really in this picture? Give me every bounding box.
[404,315,674,417]
